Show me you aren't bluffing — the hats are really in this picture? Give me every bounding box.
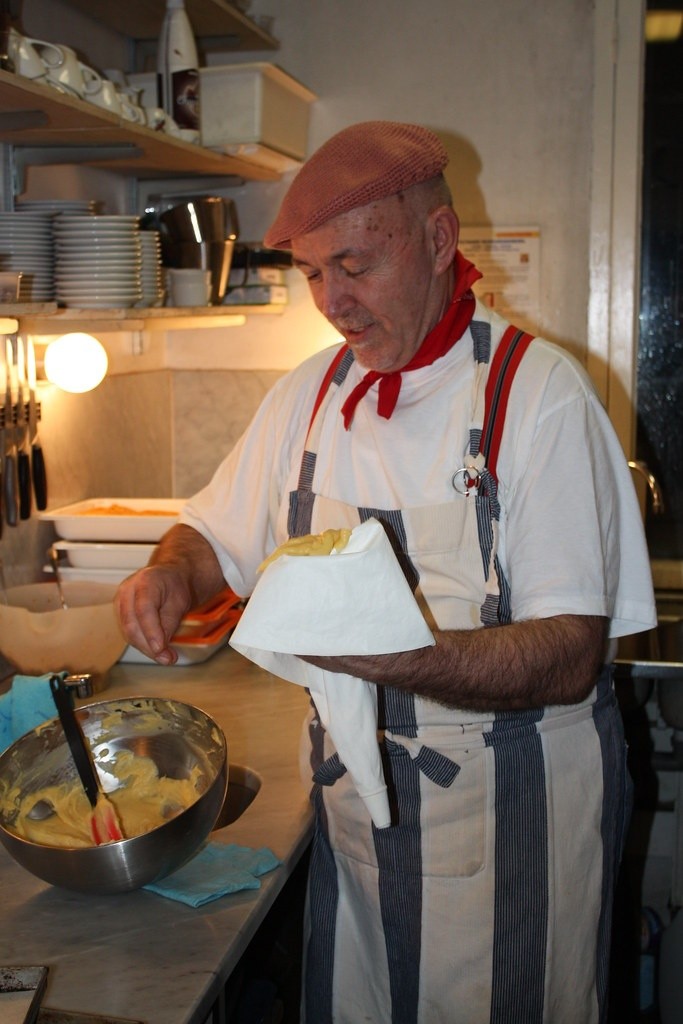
[264,122,449,251]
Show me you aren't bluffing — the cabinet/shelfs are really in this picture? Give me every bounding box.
[0,0,287,331]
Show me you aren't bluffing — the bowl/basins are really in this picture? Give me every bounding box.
[0,581,129,681]
[0,695,229,895]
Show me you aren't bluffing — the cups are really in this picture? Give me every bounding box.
[170,270,213,306]
[159,194,240,305]
[0,272,23,304]
[0,27,147,127]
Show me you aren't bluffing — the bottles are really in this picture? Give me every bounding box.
[156,0,202,146]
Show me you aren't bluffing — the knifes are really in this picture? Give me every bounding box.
[5,338,18,526]
[16,336,31,520]
[27,333,47,511]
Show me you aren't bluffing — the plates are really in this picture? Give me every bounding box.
[37,496,244,666]
[0,199,166,309]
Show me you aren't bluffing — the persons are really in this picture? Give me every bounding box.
[115,118,658,1024]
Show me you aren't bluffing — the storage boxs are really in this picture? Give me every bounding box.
[197,61,318,162]
